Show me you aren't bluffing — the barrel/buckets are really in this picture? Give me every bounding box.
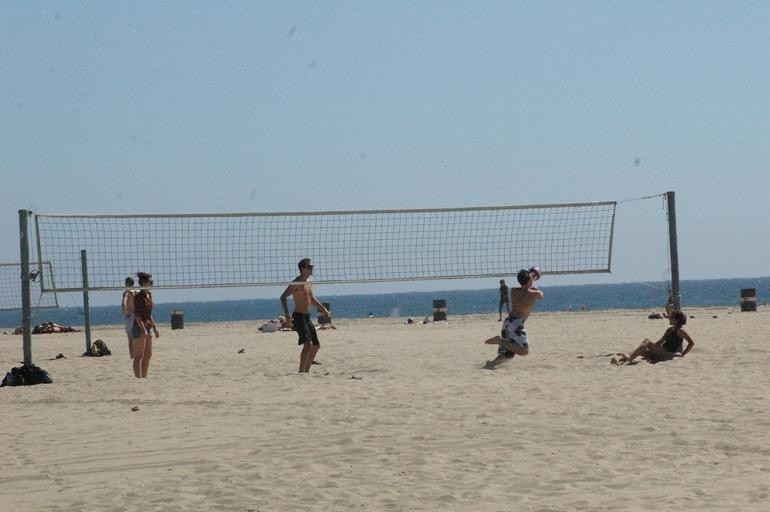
[317,302,332,324]
[740,288,756,312]
[432,299,448,322]
[170,311,185,329]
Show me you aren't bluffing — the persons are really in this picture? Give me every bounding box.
[611,311,693,365]
[31,322,81,333]
[497,279,511,321]
[483,269,543,369]
[132,272,159,378]
[122,277,140,358]
[280,258,329,373]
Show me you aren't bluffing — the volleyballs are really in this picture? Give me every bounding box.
[530,266,541,280]
